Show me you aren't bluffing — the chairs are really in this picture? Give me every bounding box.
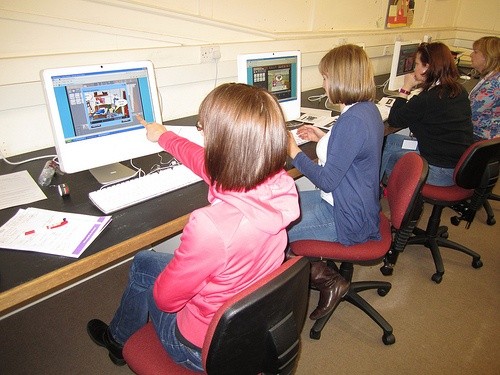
[286,152,429,345]
[383,137,500,284]
[122,255,311,375]
[481,191,500,225]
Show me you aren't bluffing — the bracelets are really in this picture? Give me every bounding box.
[400,89,410,95]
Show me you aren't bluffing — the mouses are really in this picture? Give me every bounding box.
[460,75,470,81]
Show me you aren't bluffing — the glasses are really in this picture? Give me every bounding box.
[195,120,204,131]
[420,42,431,58]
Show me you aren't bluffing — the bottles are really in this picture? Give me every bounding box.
[38,158,57,186]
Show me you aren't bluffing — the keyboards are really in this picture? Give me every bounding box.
[89,164,203,214]
[375,103,391,122]
[289,129,311,146]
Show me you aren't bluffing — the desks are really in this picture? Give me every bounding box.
[0,72,478,322]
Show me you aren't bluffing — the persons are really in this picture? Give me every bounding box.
[86,84,301,371]
[384,42,472,187]
[468,37,500,142]
[286,46,384,320]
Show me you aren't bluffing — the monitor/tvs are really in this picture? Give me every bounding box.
[237,51,301,122]
[383,41,423,96]
[40,60,166,185]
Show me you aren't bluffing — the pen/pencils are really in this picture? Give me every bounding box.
[25,218,68,235]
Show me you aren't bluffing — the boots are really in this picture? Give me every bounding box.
[285,246,351,320]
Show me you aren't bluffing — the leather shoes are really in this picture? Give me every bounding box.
[87,319,126,366]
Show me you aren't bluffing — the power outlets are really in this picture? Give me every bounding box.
[200,46,221,63]
